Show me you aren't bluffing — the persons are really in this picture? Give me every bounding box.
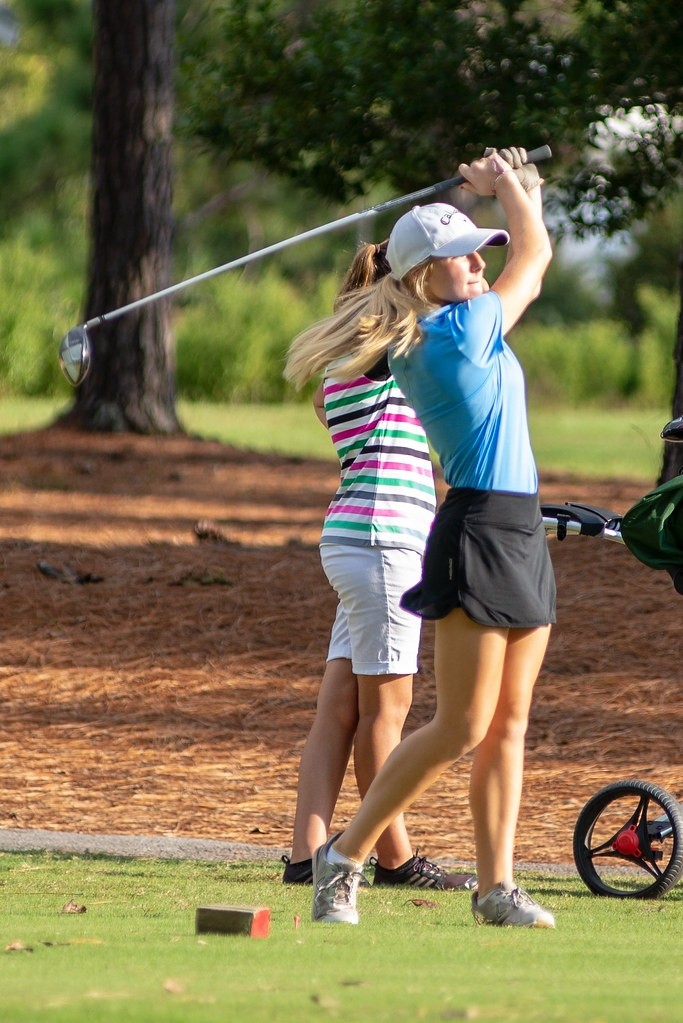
[281,146,558,930]
[279,240,479,893]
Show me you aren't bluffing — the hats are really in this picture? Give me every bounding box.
[385,202,510,282]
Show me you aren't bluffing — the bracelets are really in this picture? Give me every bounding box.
[491,161,513,190]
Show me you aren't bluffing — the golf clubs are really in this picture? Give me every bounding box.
[62,144,553,389]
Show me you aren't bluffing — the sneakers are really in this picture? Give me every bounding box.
[281,855,313,885]
[311,830,364,925]
[471,879,554,928]
[369,847,479,892]
